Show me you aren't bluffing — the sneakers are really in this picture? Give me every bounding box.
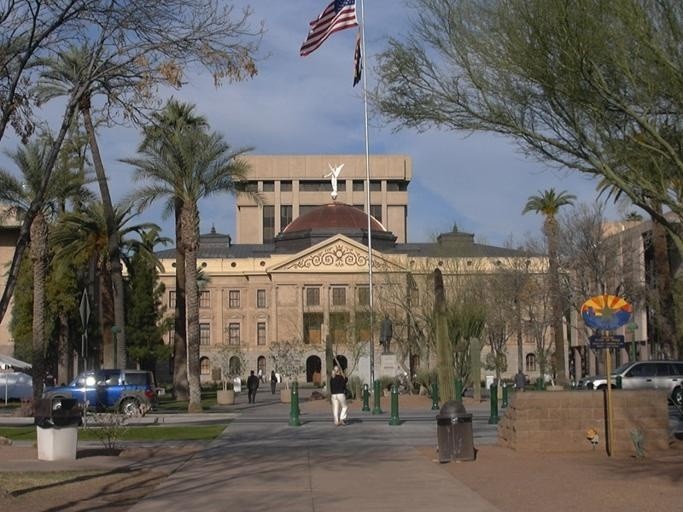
[335,419,346,426]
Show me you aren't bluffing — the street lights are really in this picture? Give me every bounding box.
[627,322,639,361]
[111,325,122,368]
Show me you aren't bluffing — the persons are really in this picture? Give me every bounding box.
[514,367,526,392]
[246,369,259,404]
[270,369,277,394]
[378,312,394,354]
[258,369,265,384]
[321,162,338,192]
[329,364,350,428]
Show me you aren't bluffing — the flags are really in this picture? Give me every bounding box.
[351,28,362,88]
[298,0,360,58]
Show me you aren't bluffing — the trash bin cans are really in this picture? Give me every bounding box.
[34,397,82,461]
[435,399,475,463]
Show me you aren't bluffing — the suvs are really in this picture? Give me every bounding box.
[41,370,158,415]
[577,358,683,404]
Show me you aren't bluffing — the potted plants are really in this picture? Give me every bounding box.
[268,338,306,402]
[210,338,249,406]
